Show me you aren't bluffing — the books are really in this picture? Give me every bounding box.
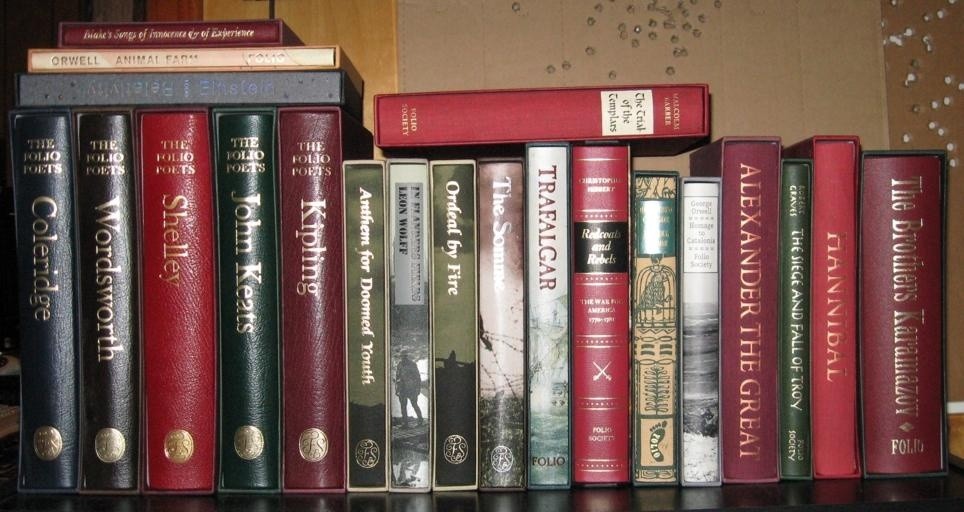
[12,20,950,502]
